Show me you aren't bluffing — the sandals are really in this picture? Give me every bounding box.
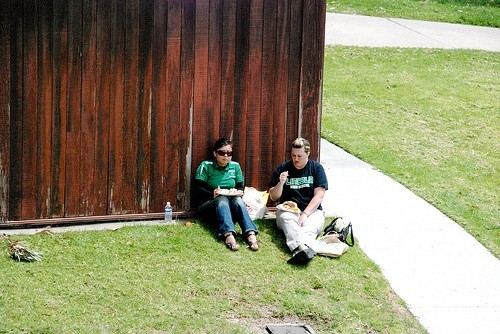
[223,233,240,252]
[246,233,259,251]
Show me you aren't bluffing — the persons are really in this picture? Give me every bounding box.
[193,138,260,251]
[268,138,328,264]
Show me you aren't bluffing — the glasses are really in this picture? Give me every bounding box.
[216,150,234,157]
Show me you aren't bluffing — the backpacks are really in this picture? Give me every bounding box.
[324,217,355,247]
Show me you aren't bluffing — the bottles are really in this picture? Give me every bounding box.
[165,202,172,223]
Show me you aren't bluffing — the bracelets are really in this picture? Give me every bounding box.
[303,211,309,217]
